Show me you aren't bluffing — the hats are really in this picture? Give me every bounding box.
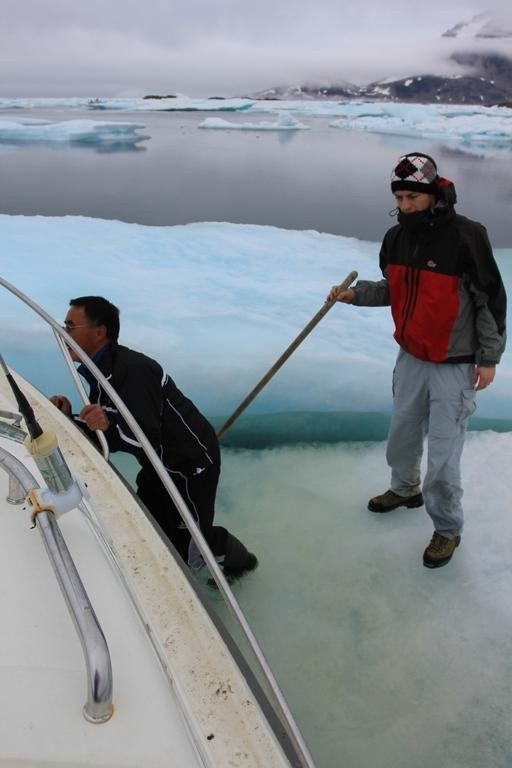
[390,153,438,195]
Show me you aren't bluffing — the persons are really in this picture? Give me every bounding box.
[49,296,259,591]
[326,152,506,568]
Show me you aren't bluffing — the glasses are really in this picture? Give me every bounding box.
[62,320,100,333]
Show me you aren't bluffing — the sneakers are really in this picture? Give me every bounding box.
[423,531,460,569]
[207,552,258,590]
[368,490,424,513]
